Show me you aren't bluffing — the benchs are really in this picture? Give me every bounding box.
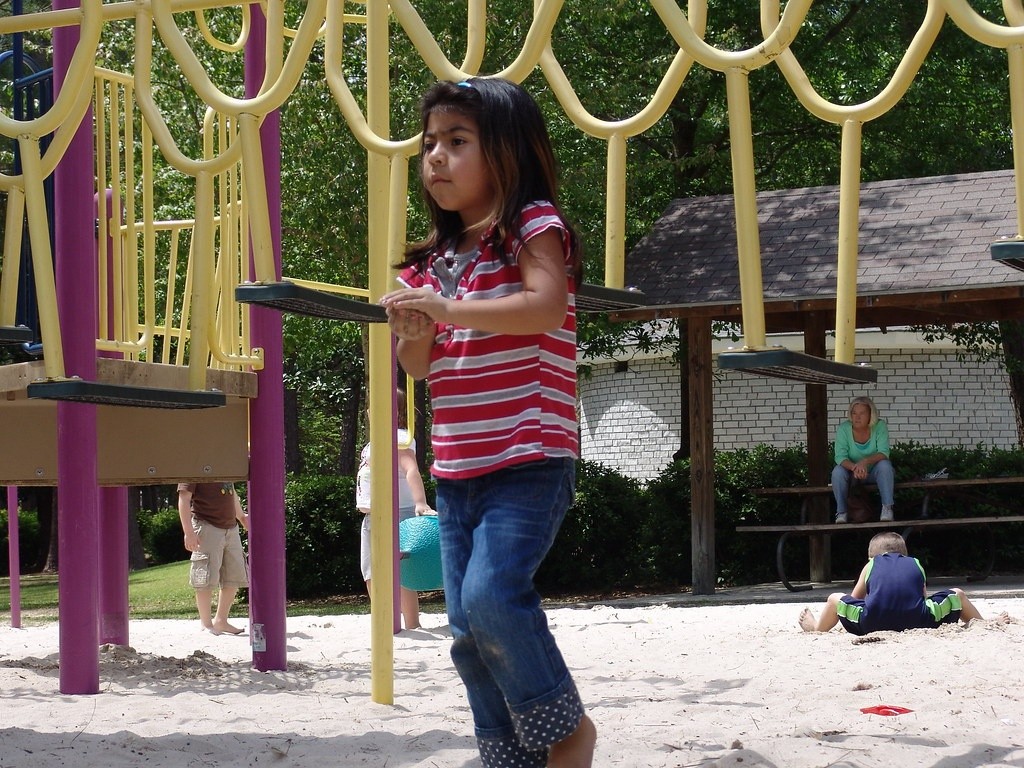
[735,476,1024,583]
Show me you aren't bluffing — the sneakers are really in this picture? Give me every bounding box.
[880,508,894,521]
[836,513,847,523]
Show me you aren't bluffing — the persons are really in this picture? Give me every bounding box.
[378,76,596,767]
[176,482,248,636]
[355,386,440,635]
[798,531,1010,633]
[830,395,894,524]
